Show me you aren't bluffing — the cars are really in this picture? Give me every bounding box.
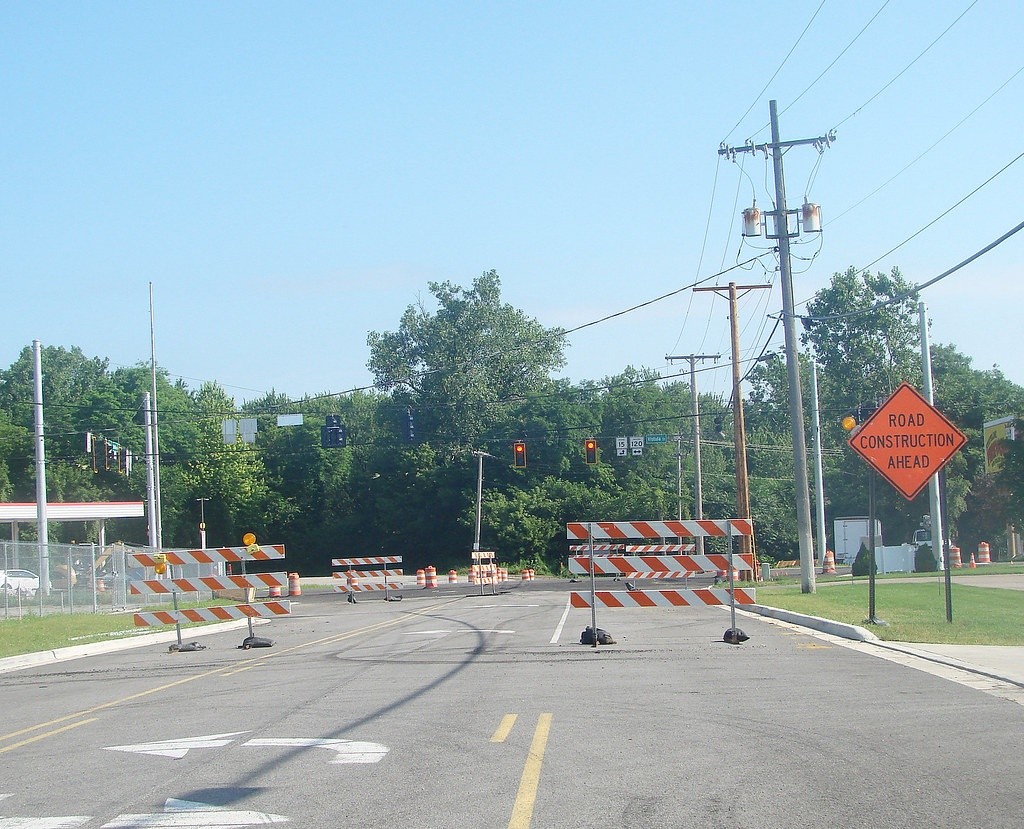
[0,569,53,590]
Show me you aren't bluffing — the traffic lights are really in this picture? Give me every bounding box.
[514,443,526,468]
[585,440,597,465]
[93,440,125,473]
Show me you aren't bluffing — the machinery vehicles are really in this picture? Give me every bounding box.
[52,540,143,591]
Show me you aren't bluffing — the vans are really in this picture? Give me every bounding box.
[911,529,951,551]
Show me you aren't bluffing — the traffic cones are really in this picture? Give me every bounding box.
[969,553,976,568]
